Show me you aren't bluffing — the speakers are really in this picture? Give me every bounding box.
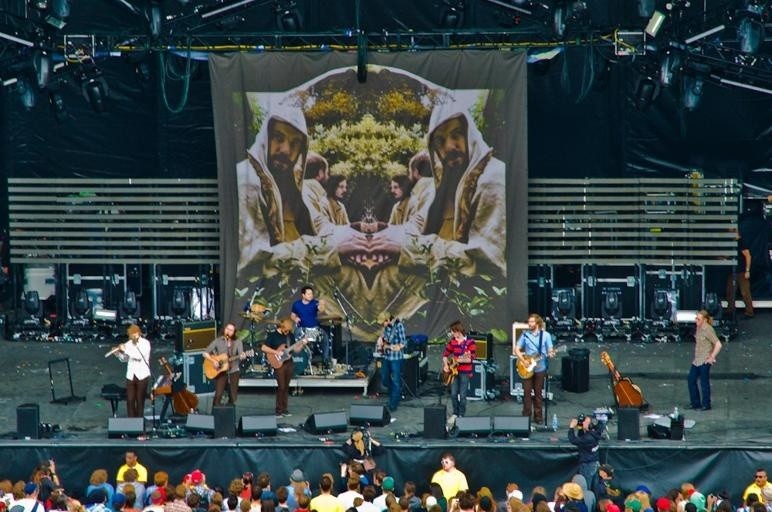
[237,415,277,434]
[214,406,236,438]
[618,408,640,440]
[185,413,214,434]
[455,416,491,437]
[107,417,145,438]
[424,406,447,438]
[350,406,391,427]
[493,416,529,436]
[16,403,39,440]
[303,411,347,435]
[561,356,589,392]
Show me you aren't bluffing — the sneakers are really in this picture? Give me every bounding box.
[275,413,282,418]
[282,410,291,417]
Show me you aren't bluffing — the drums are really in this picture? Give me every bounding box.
[295,327,322,345]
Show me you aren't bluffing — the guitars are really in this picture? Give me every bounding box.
[516,345,566,379]
[267,339,307,368]
[204,349,254,379]
[377,339,402,355]
[443,355,472,386]
[160,357,198,413]
[601,352,642,408]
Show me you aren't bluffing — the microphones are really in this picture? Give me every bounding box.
[227,334,229,340]
[133,336,136,344]
[333,290,338,300]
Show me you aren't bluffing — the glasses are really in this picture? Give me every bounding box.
[441,460,450,465]
[755,475,763,478]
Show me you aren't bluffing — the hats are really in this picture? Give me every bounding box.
[508,490,522,500]
[690,491,706,509]
[382,477,394,489]
[291,469,303,482]
[562,483,583,500]
[598,463,614,476]
[192,472,203,483]
[761,488,772,500]
[24,484,36,493]
[605,498,670,512]
[112,494,126,505]
[477,487,492,499]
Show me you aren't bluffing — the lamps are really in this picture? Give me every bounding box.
[492,412,533,441]
[447,413,490,443]
[103,401,390,441]
[1,0,772,121]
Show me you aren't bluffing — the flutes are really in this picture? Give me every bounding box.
[105,339,132,357]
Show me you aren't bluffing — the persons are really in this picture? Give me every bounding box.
[233,94,508,325]
[261,319,305,419]
[567,415,604,490]
[374,312,405,409]
[718,225,755,320]
[202,323,247,412]
[289,282,333,371]
[112,323,152,419]
[684,310,723,412]
[443,320,477,422]
[1,425,772,512]
[513,313,557,425]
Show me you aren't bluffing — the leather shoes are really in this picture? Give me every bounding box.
[684,404,695,409]
[701,406,709,410]
